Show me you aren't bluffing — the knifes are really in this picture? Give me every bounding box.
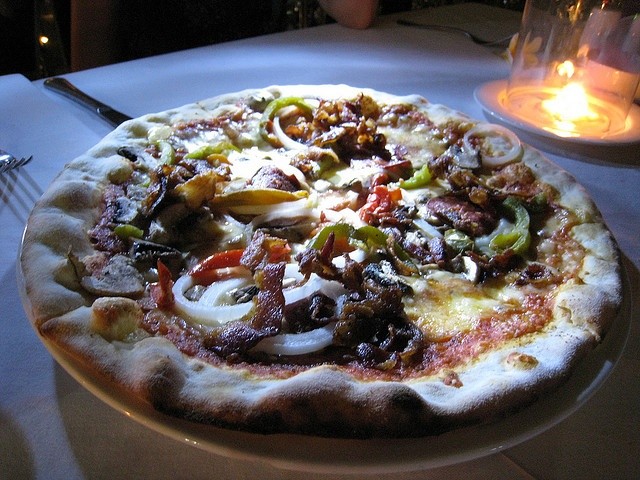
[43,78,133,128]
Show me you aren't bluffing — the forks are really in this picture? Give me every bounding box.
[0,151,33,171]
[395,20,521,47]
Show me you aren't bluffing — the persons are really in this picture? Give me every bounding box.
[316,0,378,32]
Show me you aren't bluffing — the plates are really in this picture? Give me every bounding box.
[474,79,639,144]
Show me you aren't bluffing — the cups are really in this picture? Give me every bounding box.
[507,1,640,139]
